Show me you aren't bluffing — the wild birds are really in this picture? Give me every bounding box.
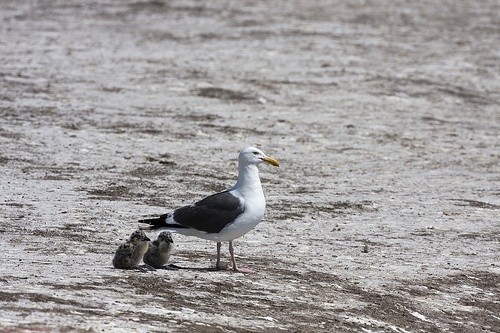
[137,147,280,274]
[142,231,179,272]
[111,228,147,274]
